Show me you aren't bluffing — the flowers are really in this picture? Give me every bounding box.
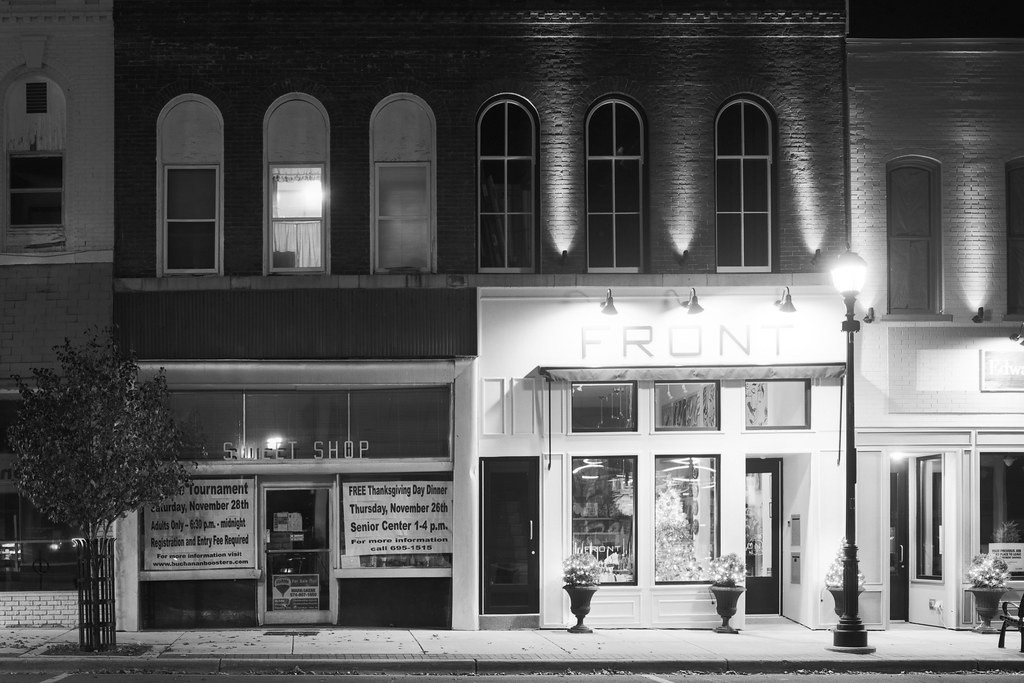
[563,552,603,587]
[967,552,1011,587]
[709,552,748,588]
[825,534,866,589]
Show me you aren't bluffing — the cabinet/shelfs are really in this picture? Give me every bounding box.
[573,518,631,573]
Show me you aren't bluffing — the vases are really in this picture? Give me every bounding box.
[563,585,600,634]
[709,586,748,633]
[828,588,866,618]
[965,587,1010,634]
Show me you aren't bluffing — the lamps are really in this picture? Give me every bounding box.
[863,308,875,323]
[677,250,690,266]
[680,288,704,316]
[600,288,618,315]
[1002,454,1015,466]
[972,307,984,323]
[558,250,569,265]
[774,286,797,312]
[811,249,821,265]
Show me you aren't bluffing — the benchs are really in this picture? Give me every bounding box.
[998,593,1024,652]
